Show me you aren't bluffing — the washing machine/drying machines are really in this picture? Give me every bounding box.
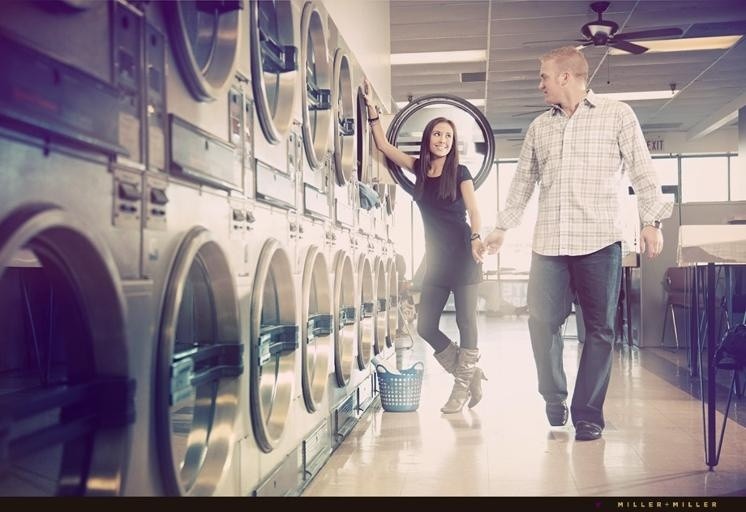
[0,0,496,497]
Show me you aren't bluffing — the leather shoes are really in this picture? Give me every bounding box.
[575,420,602,440]
[546,400,568,426]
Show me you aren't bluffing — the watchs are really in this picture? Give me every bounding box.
[468,232,482,243]
[640,221,663,230]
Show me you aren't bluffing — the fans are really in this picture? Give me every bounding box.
[521,1,686,55]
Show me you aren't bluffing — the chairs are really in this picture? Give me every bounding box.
[659,220,746,472]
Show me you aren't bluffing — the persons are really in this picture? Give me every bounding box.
[482,46,675,441]
[362,79,488,414]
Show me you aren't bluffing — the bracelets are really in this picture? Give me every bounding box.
[368,116,379,122]
[496,226,507,232]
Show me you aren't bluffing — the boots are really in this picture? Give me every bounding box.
[434,339,488,413]
